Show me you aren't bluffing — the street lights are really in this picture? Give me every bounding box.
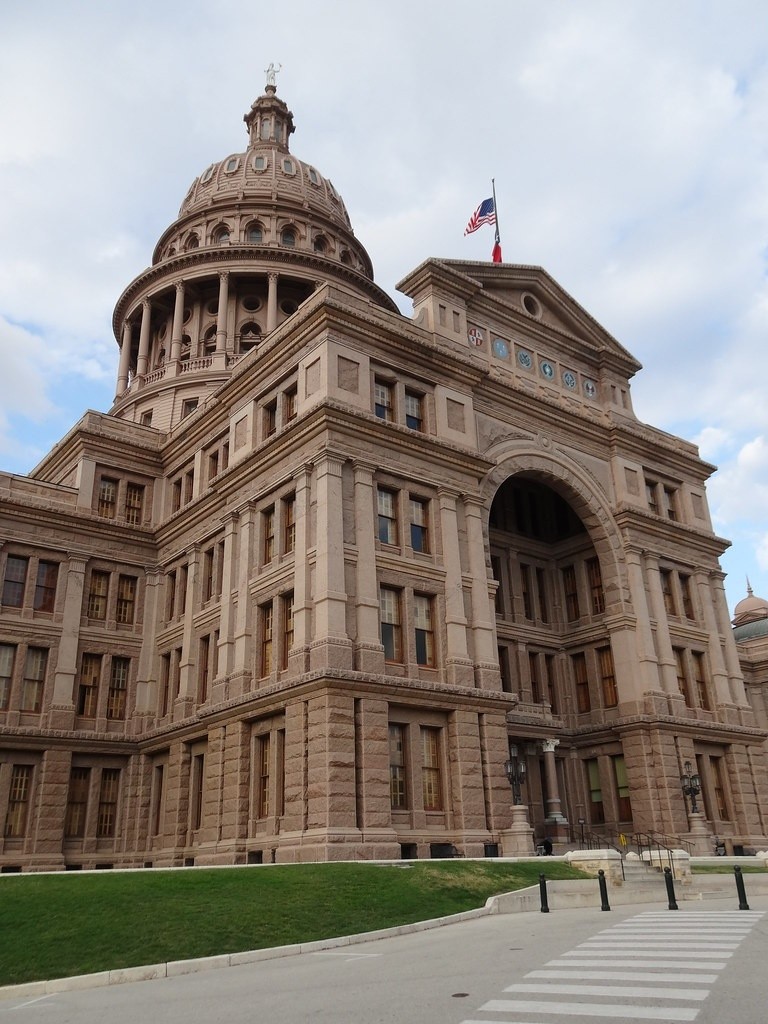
[504,744,527,806]
[681,761,703,814]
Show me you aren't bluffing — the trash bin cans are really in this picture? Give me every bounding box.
[733,844,744,856]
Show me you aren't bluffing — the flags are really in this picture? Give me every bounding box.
[462,196,496,238]
